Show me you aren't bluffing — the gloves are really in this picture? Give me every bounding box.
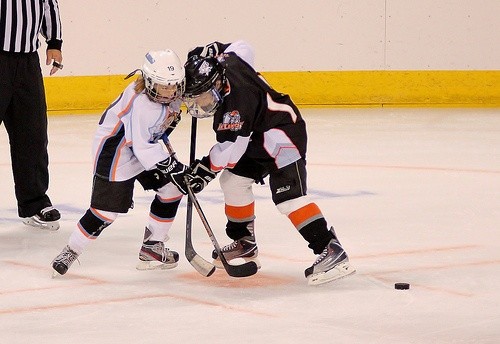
[186,158,217,193]
[154,155,189,197]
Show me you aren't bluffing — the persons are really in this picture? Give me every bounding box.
[50,49,191,275]
[0,0,63,223]
[184,42,348,280]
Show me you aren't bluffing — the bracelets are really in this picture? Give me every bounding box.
[52,62,63,70]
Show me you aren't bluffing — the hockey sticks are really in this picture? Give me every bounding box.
[184,108,216,278]
[164,138,259,277]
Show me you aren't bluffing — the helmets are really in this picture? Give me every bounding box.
[185,45,227,119]
[142,48,186,104]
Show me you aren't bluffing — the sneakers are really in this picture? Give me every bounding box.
[212,222,261,272]
[20,204,61,231]
[303,226,354,285]
[137,243,180,273]
[50,245,81,281]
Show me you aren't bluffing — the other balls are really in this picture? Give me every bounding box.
[394,283,411,290]
